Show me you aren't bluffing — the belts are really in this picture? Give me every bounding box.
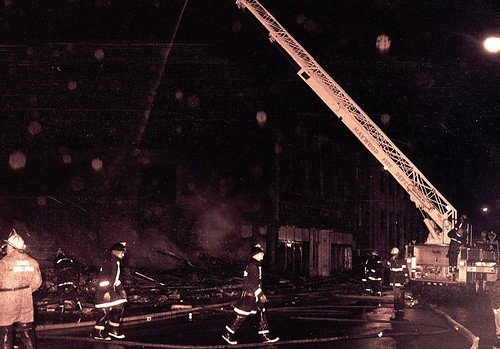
[0,286,29,291]
[245,293,254,296]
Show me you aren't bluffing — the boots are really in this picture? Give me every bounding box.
[258,330,279,343]
[108,320,125,338]
[75,302,82,311]
[58,305,65,313]
[94,325,111,340]
[390,310,404,321]
[222,325,238,344]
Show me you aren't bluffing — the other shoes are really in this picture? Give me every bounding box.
[378,292,382,297]
[371,291,375,295]
[454,268,459,272]
[449,268,453,273]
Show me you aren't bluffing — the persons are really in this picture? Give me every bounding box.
[448,228,466,282]
[93,241,126,340]
[455,214,467,231]
[54,247,84,312]
[386,247,408,320]
[361,250,383,296]
[0,233,43,349]
[222,244,280,345]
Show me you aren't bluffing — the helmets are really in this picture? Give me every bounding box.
[372,251,378,256]
[250,244,264,256]
[109,241,127,251]
[4,229,26,249]
[390,247,399,254]
[456,228,463,237]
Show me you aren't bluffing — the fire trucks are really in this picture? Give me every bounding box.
[233,0,500,308]
